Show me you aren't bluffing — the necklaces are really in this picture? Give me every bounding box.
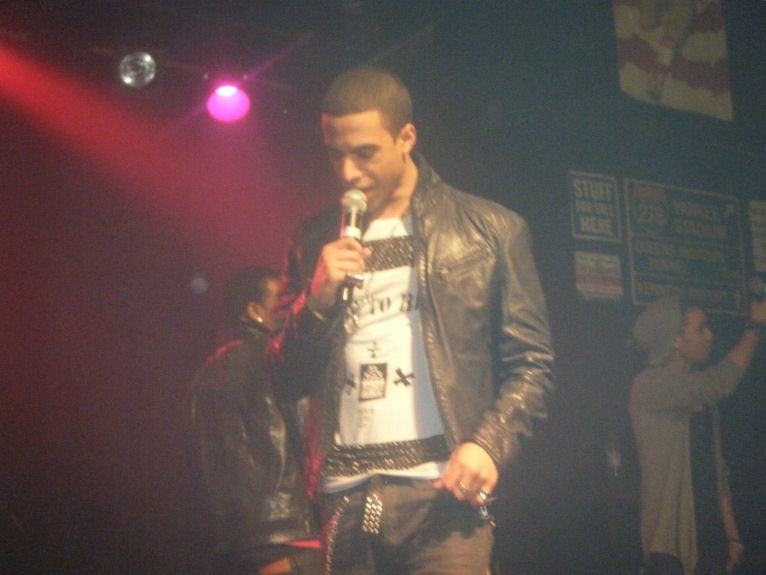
[343,216,401,335]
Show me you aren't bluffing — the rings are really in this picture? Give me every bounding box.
[457,481,469,493]
[347,273,363,284]
[477,490,489,499]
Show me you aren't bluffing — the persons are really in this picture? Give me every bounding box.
[187,265,324,575]
[628,291,766,575]
[267,69,554,575]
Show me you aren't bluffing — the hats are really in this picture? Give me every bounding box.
[633,296,683,365]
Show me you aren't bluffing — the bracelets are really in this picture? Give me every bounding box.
[746,320,766,335]
[730,538,742,544]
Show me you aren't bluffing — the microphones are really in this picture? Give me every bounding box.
[337,190,368,305]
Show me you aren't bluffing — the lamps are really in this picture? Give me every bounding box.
[117,47,158,91]
[203,67,251,125]
